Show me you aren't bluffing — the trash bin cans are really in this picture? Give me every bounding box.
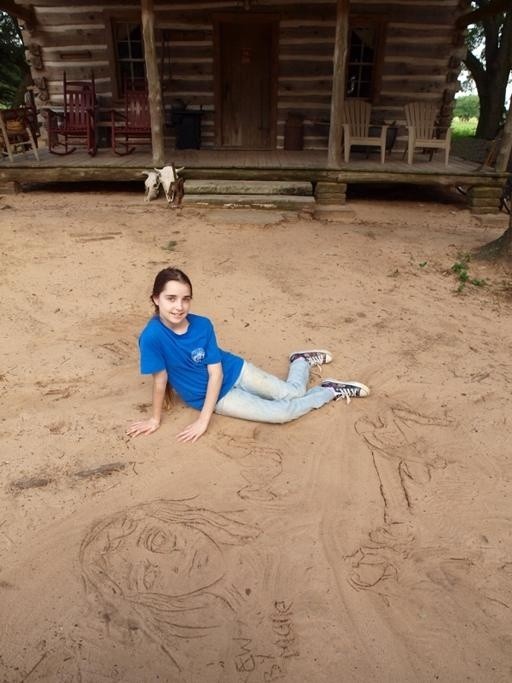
[284,111,305,151]
[175,111,205,150]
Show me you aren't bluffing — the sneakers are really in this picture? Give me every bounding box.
[289,350,333,365]
[321,378,370,405]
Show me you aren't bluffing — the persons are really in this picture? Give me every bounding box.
[123,265,373,446]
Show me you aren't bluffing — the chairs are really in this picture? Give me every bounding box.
[400,98,453,169]
[338,96,388,164]
[1,67,169,161]
